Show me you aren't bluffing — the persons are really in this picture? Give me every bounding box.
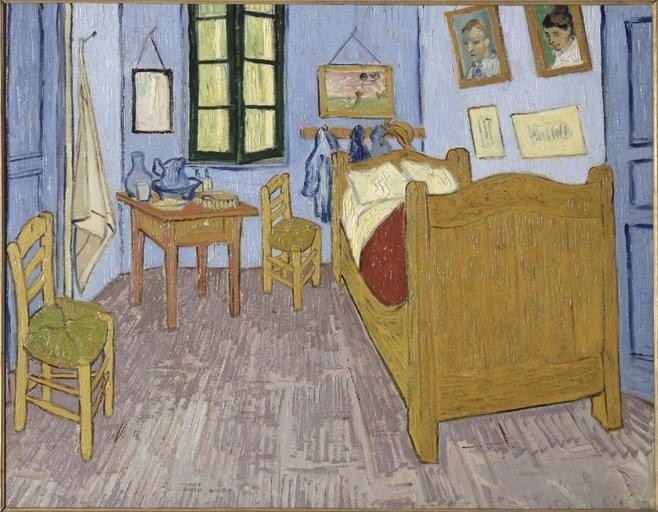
[461,18,500,79]
[540,5,582,69]
